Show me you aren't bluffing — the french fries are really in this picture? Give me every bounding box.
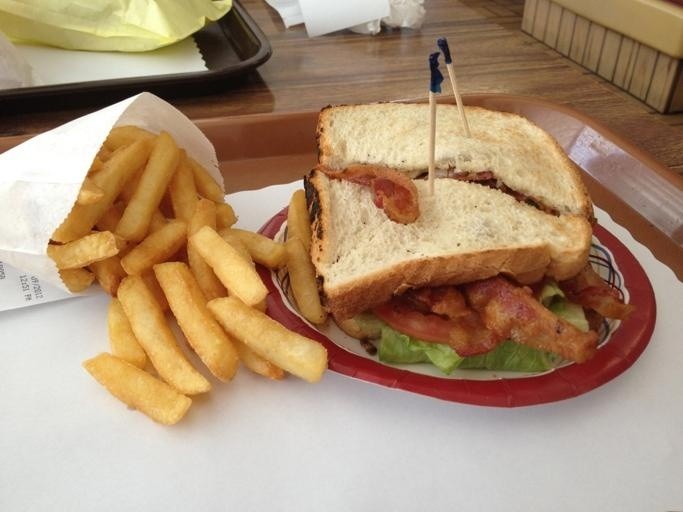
[47,123,328,426]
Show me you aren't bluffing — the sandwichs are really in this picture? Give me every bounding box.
[307,99,639,371]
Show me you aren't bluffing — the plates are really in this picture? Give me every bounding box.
[253,190,657,408]
